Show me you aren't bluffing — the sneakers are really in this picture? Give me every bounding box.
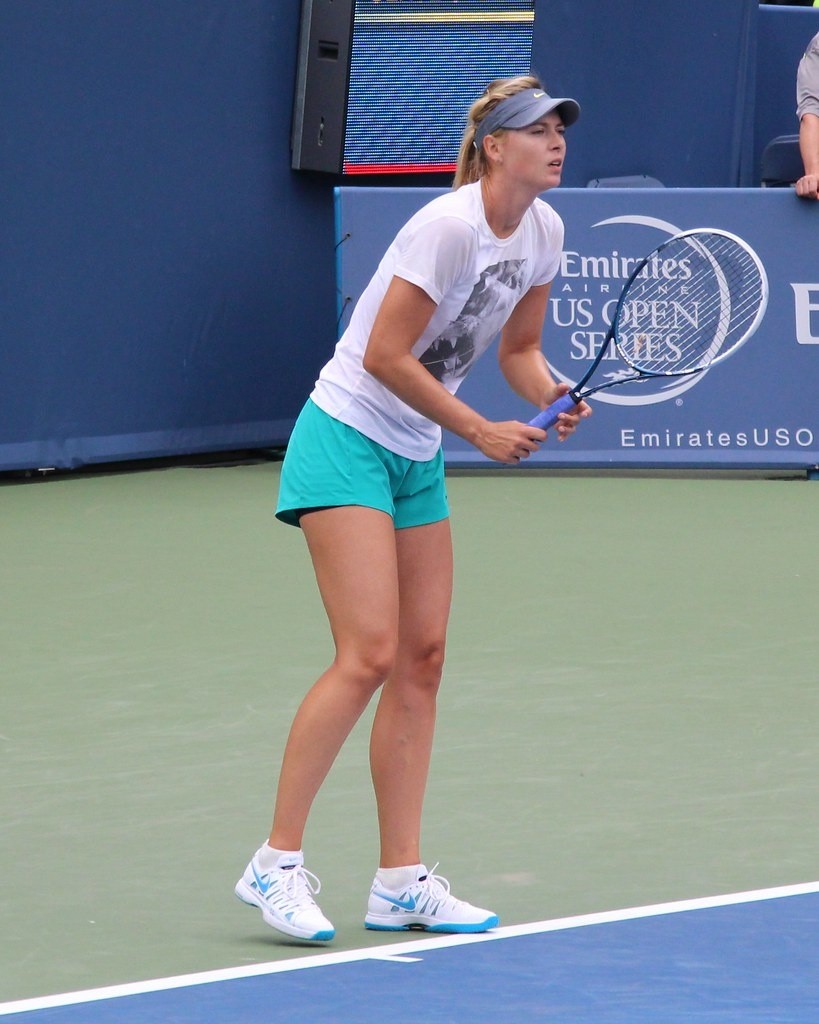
[365,874,499,934]
[234,850,332,940]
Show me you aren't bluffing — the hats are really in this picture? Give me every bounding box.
[471,88,581,152]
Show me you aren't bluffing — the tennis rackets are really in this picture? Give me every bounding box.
[503,226,768,484]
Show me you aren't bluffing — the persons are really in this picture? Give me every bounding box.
[233,74,592,941]
[794,29,819,201]
